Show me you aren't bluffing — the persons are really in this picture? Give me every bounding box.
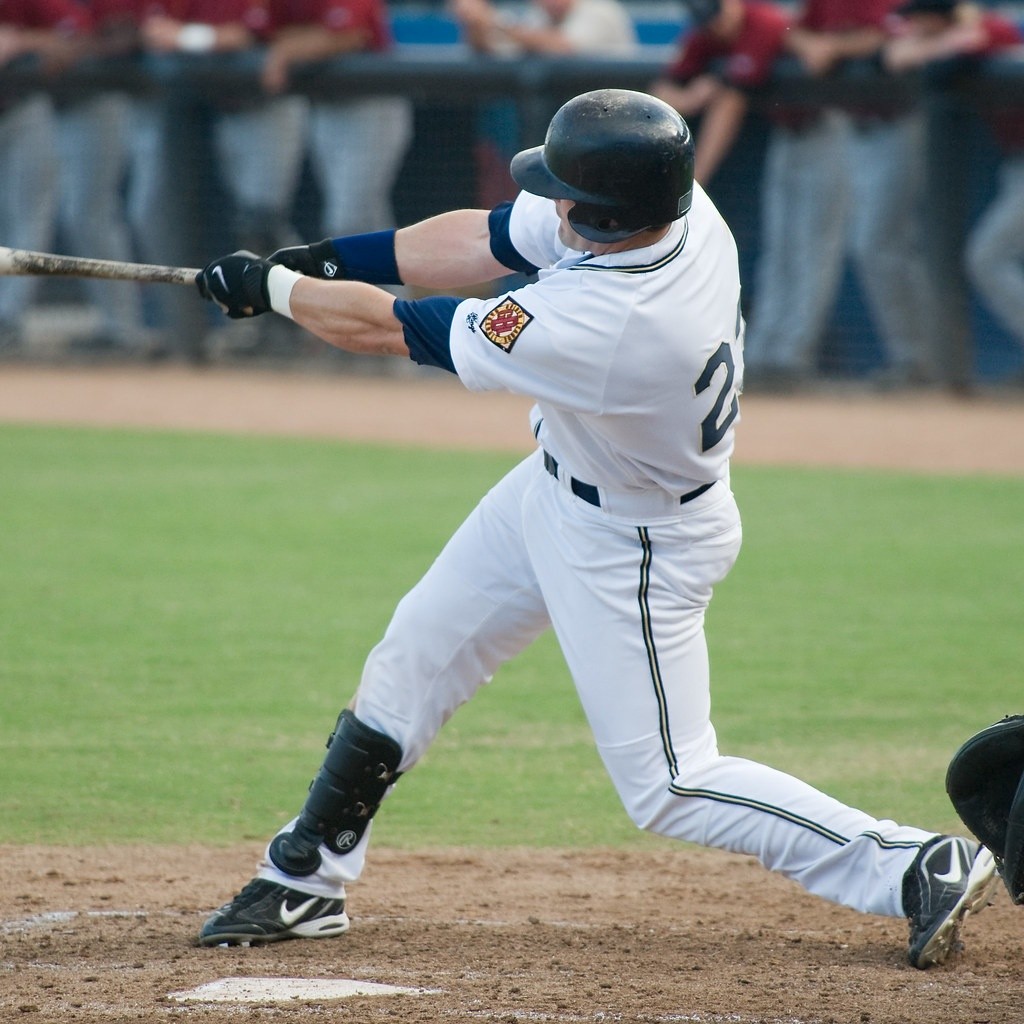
[0,0,1023,384]
[192,89,998,970]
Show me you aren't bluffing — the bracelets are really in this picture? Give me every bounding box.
[174,21,218,55]
[265,262,304,322]
[320,231,403,285]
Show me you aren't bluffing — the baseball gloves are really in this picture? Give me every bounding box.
[945,714,1024,906]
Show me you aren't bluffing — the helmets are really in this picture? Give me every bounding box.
[510,88,695,244]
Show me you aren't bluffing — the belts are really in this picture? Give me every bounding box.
[543,448,718,508]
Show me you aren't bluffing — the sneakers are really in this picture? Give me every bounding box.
[198,877,350,949]
[902,834,1002,969]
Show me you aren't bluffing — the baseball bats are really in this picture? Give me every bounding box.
[0,244,200,286]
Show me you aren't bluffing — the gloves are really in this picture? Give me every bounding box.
[195,250,277,320]
[266,237,353,282]
